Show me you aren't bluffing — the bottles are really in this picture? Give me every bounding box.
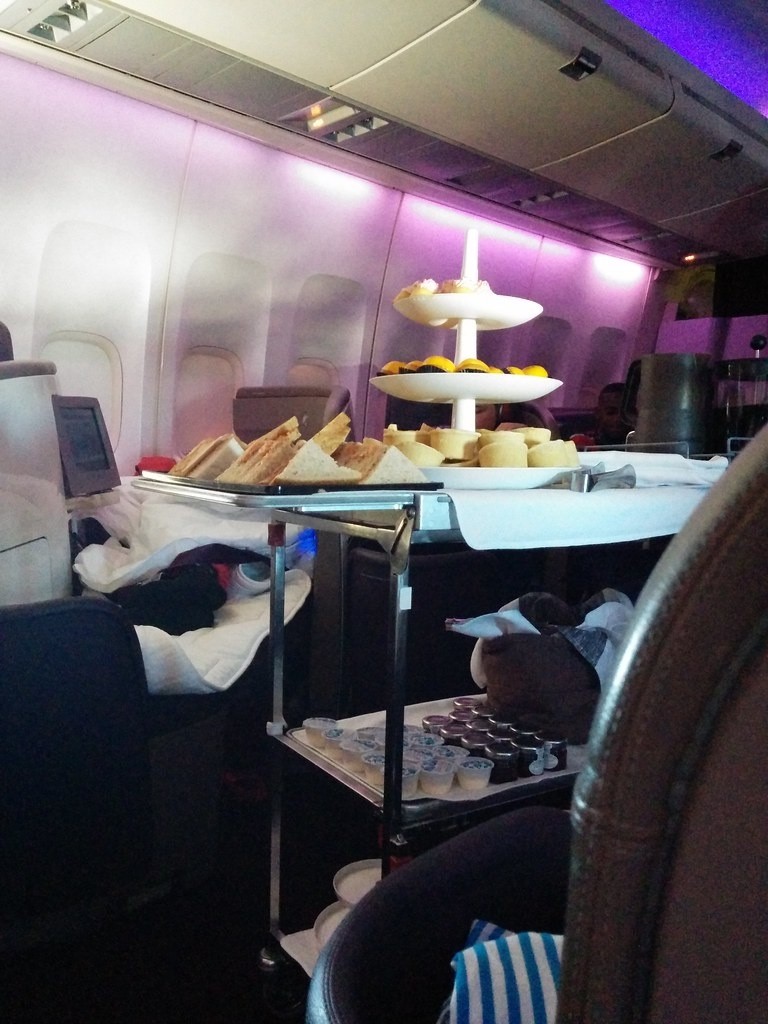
[303,695,568,791]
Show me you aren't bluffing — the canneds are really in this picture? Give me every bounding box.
[421,695,568,781]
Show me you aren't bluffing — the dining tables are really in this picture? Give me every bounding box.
[168,484,715,1006]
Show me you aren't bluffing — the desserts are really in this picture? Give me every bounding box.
[376,278,581,470]
[302,716,493,796]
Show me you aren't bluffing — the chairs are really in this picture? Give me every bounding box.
[310,426,765,1023]
[231,377,357,453]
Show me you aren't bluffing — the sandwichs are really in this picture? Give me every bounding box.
[169,413,431,486]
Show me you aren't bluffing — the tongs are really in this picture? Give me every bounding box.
[570,461,637,493]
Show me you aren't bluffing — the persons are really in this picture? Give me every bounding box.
[475,403,503,432]
[720,383,747,408]
[567,381,628,451]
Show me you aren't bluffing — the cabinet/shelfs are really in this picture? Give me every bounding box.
[92,0,766,262]
